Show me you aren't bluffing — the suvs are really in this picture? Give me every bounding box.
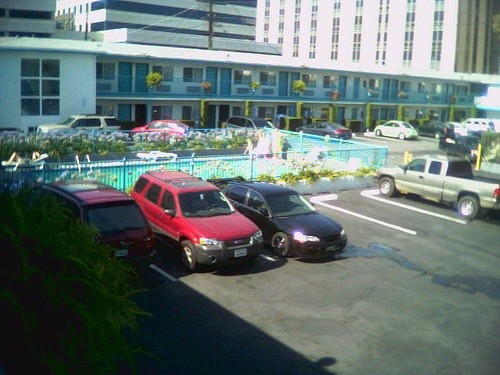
[36,114,123,139]
[126,169,264,273]
[221,115,277,131]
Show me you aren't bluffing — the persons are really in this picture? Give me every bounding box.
[270,127,283,158]
[438,126,455,149]
[244,137,253,155]
[253,131,271,156]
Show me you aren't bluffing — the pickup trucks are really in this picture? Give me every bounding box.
[373,153,500,221]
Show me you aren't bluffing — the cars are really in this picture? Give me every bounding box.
[416,117,500,164]
[130,120,193,142]
[373,120,419,140]
[296,121,352,142]
[26,179,158,265]
[213,176,348,261]
